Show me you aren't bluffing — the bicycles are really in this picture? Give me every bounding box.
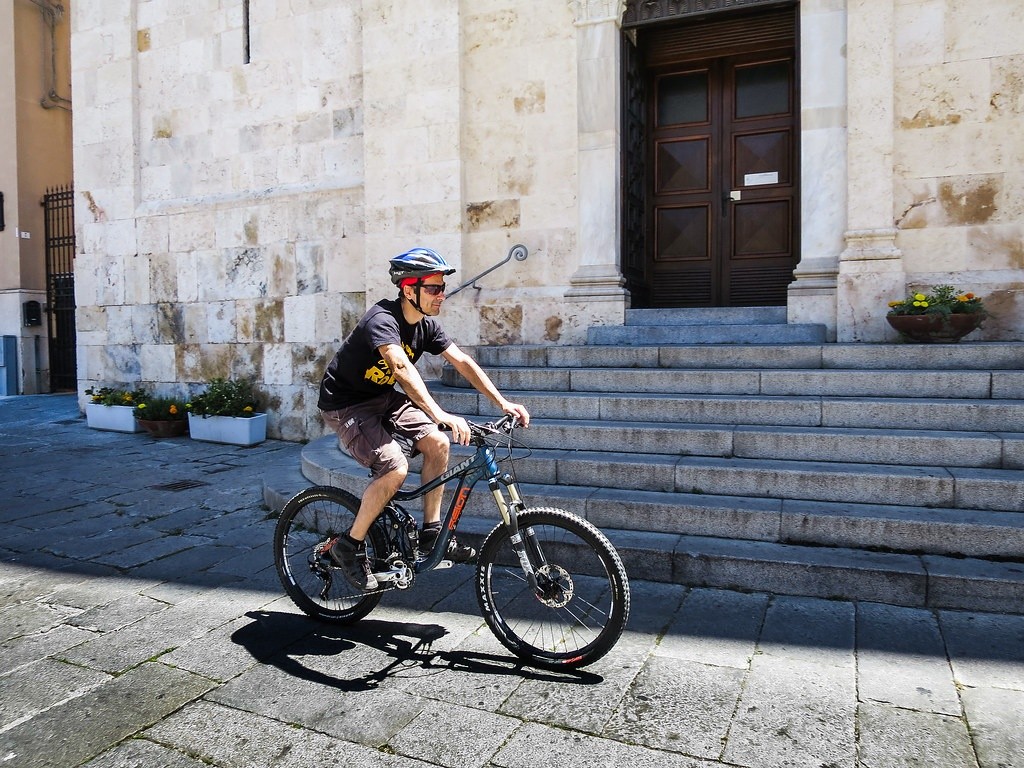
[272,413,633,675]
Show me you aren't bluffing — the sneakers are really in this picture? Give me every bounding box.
[420,525,477,562]
[330,529,378,592]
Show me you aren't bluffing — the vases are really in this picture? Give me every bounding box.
[187,411,268,447]
[136,418,190,438]
[84,402,144,434]
[886,313,986,344]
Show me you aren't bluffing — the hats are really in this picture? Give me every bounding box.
[400,272,444,288]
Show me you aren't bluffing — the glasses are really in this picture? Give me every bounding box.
[412,283,446,296]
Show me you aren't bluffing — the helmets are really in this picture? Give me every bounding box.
[389,247,456,288]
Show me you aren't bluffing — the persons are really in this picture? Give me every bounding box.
[317,247,531,589]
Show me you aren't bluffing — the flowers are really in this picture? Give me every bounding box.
[132,394,189,423]
[887,283,999,332]
[84,385,155,408]
[184,376,261,419]
